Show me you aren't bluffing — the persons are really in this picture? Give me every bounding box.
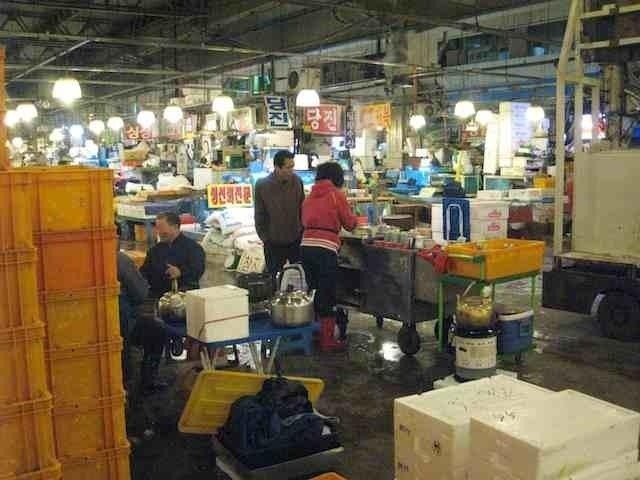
[300,161,360,353]
[114,224,167,395]
[138,214,207,324]
[252,149,306,276]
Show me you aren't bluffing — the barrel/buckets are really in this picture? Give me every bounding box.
[454,328,497,381]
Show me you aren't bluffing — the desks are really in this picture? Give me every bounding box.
[128,290,313,382]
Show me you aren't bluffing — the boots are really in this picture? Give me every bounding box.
[142,363,170,394]
[319,317,345,352]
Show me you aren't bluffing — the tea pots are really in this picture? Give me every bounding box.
[454,279,497,329]
[157,279,187,326]
[264,264,318,329]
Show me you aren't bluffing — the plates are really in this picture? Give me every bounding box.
[384,215,415,230]
[376,231,408,249]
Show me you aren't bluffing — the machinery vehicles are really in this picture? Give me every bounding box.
[542,0,640,341]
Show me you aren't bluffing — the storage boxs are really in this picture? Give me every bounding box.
[210,434,344,479]
[470,388,638,479]
[468,202,510,219]
[392,374,555,479]
[470,220,508,236]
[185,285,249,342]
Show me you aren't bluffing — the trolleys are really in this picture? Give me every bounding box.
[327,230,481,359]
[433,252,537,362]
